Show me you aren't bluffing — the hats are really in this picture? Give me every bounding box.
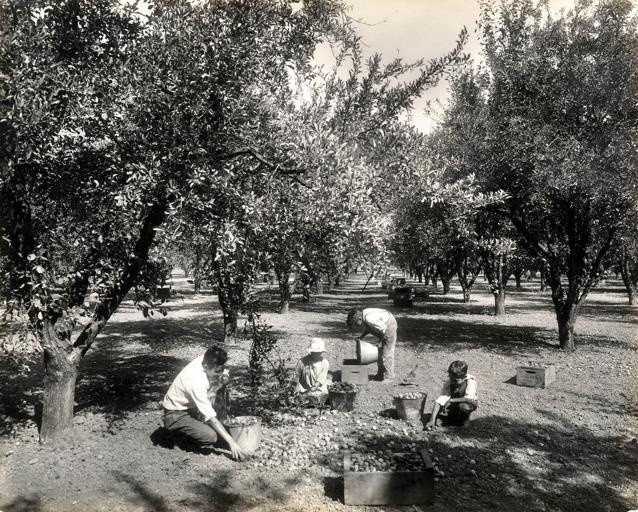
[305,337,327,353]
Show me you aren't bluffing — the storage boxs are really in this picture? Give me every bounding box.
[516,360,555,388]
[341,359,368,385]
[343,449,433,505]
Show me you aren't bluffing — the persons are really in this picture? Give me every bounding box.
[288,337,329,408]
[426,360,478,430]
[163,347,246,462]
[347,308,398,385]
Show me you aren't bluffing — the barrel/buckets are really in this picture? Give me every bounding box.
[357,339,379,364]
